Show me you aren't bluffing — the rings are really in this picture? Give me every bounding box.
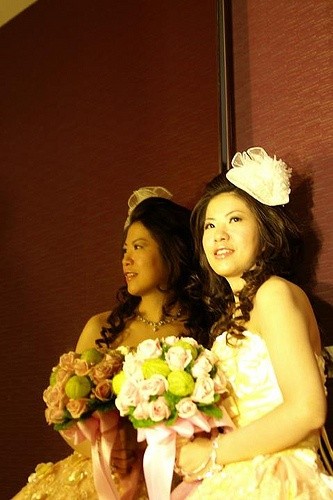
[111,460,114,466]
[112,467,117,473]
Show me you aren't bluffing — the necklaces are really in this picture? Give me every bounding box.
[136,306,184,332]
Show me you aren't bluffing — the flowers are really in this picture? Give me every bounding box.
[230,145,291,209]
[123,186,172,230]
[44,349,122,432]
[112,334,234,445]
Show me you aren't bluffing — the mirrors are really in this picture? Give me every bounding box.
[0,0,238,500]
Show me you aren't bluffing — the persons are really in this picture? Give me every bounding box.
[174,171,333,500]
[10,197,208,500]
